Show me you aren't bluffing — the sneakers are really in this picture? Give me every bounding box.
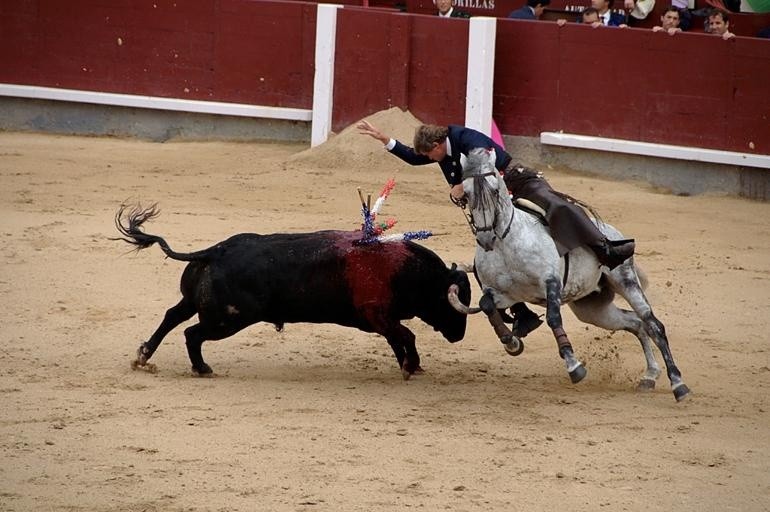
[513,313,538,336]
[608,241,635,269]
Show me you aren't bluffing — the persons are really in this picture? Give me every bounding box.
[433,0,471,18]
[576,0,631,29]
[557,8,604,28]
[358,116,636,337]
[507,1,552,22]
[624,0,770,41]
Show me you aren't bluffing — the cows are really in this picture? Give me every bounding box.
[110,202,472,381]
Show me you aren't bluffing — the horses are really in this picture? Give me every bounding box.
[454,146,693,403]
